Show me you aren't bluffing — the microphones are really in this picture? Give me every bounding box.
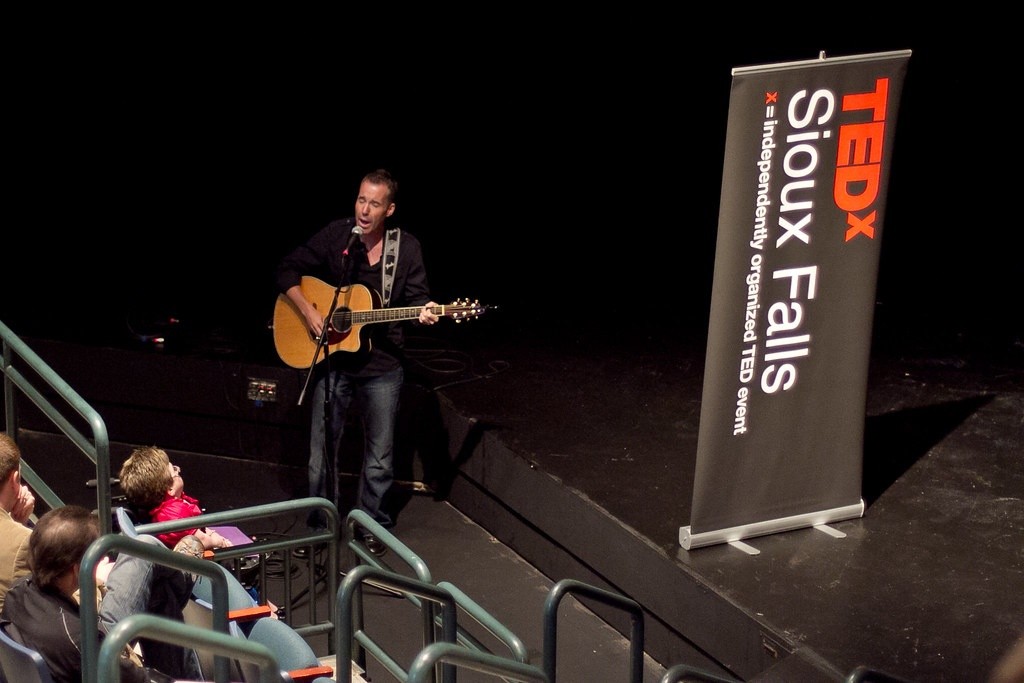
[342,226,364,257]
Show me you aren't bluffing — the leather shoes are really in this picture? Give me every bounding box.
[352,524,387,557]
[292,528,329,557]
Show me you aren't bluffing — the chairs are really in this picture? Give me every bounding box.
[1,498,349,682]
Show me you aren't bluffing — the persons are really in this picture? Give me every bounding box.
[0,432,235,683]
[275,168,439,557]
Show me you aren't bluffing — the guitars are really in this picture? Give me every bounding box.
[272,274,499,372]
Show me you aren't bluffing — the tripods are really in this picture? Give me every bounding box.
[279,247,405,614]
